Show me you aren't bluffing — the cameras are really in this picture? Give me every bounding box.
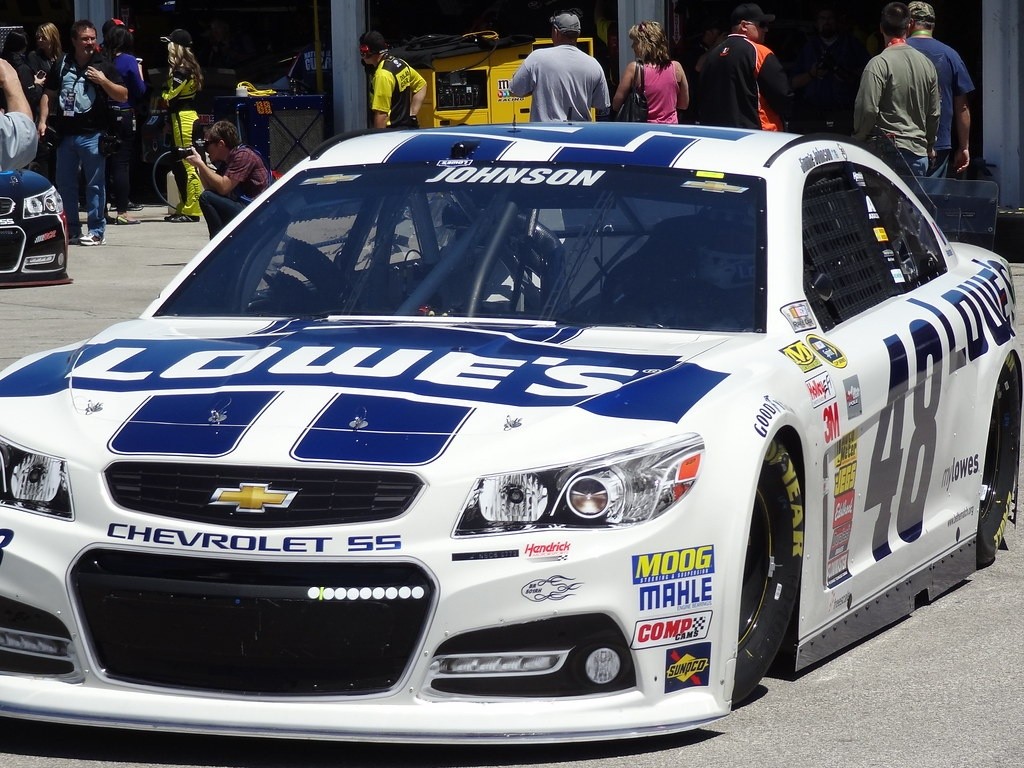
[172,139,207,163]
[98,136,122,156]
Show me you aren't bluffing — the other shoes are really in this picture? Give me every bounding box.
[164,213,200,222]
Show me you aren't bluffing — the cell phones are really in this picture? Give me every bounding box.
[36,70,46,79]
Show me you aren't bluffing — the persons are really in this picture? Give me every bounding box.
[852,1,975,194]
[786,5,870,111]
[0,18,152,225]
[184,121,270,241]
[688,3,785,133]
[160,29,205,223]
[201,17,246,70]
[359,30,427,128]
[613,21,689,124]
[507,13,611,122]
[37,19,128,246]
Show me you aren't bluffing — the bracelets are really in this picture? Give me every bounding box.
[808,70,814,79]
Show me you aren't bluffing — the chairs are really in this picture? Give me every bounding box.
[630,210,753,333]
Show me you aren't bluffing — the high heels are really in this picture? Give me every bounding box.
[105,216,116,223]
[116,216,142,224]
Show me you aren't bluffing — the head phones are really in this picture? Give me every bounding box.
[359,32,371,54]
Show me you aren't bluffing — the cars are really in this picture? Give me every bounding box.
[0,169,74,286]
[0,121,1022,745]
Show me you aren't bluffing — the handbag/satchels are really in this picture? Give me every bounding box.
[615,61,648,122]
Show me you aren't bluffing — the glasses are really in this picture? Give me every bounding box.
[204,138,218,145]
[747,20,767,28]
[554,9,580,20]
[639,22,651,38]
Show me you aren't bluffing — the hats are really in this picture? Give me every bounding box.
[160,28,193,47]
[729,3,776,27]
[907,1,936,22]
[553,12,582,32]
[699,15,729,29]
[102,18,134,37]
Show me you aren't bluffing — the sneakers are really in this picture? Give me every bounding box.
[107,199,144,210]
[64,229,84,244]
[78,232,106,245]
